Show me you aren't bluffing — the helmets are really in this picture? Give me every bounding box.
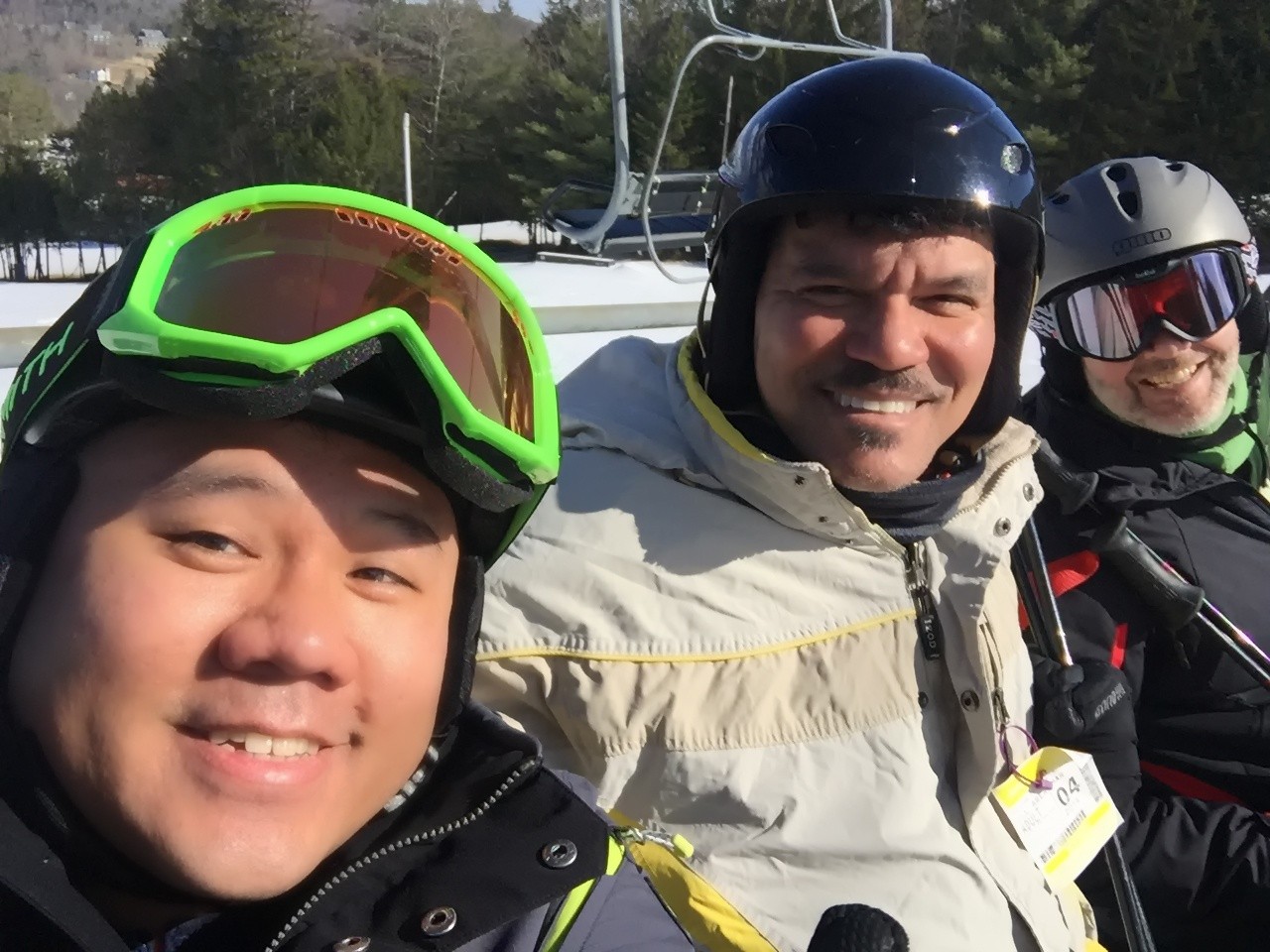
[1033,155,1257,302]
[701,55,1047,298]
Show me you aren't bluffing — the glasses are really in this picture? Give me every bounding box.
[98,182,563,483]
[1036,248,1250,361]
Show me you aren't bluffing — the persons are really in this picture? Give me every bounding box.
[0,183,702,952]
[1012,156,1270,952]
[470,58,1096,952]
